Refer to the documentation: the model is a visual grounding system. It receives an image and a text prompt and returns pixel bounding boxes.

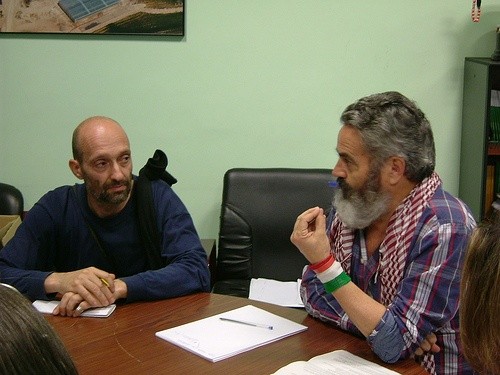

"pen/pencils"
[101,278,112,289]
[220,318,274,330]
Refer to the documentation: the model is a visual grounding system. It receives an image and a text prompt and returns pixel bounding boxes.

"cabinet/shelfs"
[460,57,500,221]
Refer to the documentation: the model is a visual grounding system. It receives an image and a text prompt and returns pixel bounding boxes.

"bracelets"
[307,253,351,294]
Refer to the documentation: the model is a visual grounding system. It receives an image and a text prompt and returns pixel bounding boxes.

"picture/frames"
[0,0,184,36]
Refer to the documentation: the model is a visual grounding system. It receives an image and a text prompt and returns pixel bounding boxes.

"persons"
[458,211,500,375]
[0,285,78,375]
[0,116,211,318]
[290,91,478,375]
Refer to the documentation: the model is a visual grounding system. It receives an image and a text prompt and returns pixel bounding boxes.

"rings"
[76,306,84,313]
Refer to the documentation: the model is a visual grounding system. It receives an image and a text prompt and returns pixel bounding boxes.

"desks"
[42,292,433,375]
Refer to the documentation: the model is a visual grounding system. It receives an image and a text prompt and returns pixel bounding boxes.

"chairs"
[199,167,338,298]
